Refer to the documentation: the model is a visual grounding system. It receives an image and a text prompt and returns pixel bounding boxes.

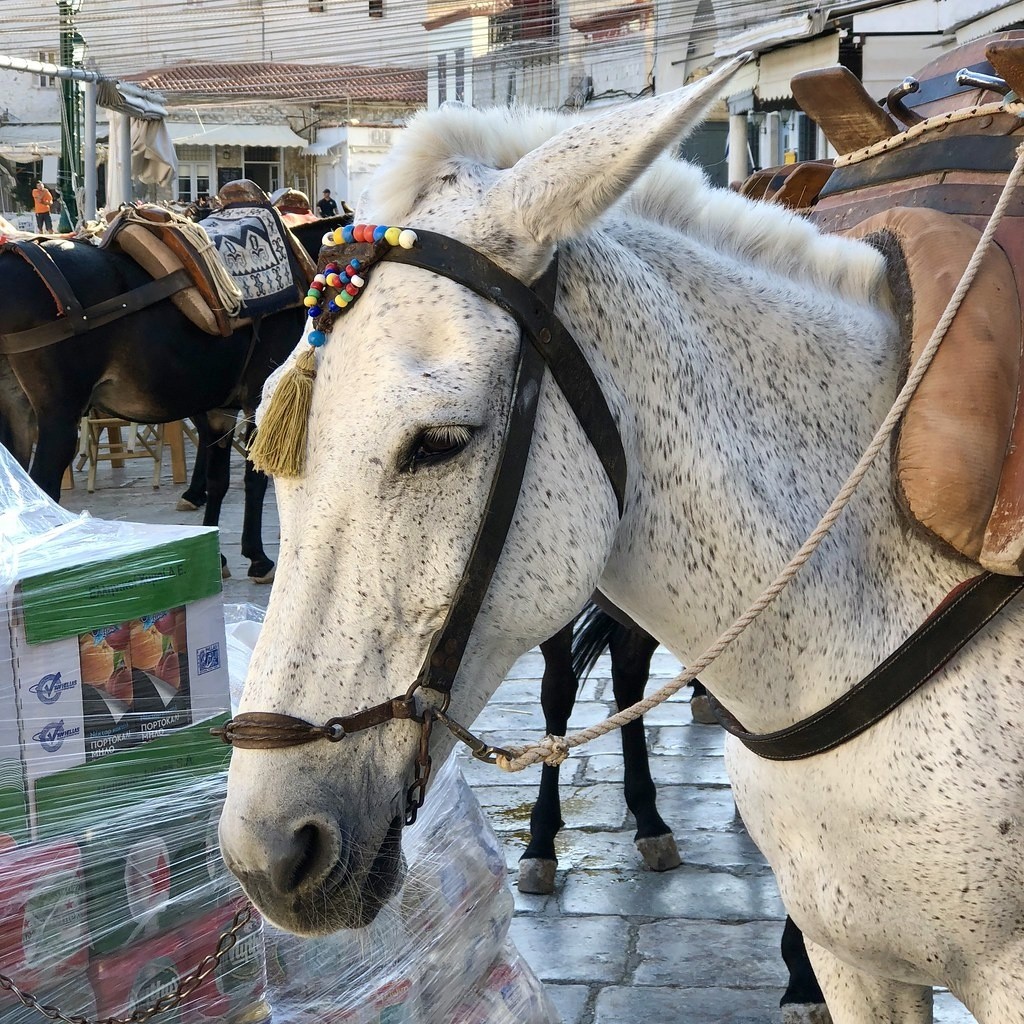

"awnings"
[300,139,346,158]
[164,122,308,150]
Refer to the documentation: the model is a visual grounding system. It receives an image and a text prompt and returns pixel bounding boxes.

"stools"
[78,405,168,493]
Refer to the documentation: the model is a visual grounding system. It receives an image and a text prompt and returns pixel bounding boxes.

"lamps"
[223,145,232,160]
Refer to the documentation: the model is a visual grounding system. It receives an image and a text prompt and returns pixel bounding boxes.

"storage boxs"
[0,521,271,1024]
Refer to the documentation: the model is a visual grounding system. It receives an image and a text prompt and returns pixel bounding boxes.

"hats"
[323,189,330,193]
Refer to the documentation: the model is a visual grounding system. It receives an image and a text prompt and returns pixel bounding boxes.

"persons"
[32,180,53,235]
[317,189,339,219]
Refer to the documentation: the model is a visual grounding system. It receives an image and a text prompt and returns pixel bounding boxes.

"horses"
[215,50,1024,1024]
[1,201,834,1023]
